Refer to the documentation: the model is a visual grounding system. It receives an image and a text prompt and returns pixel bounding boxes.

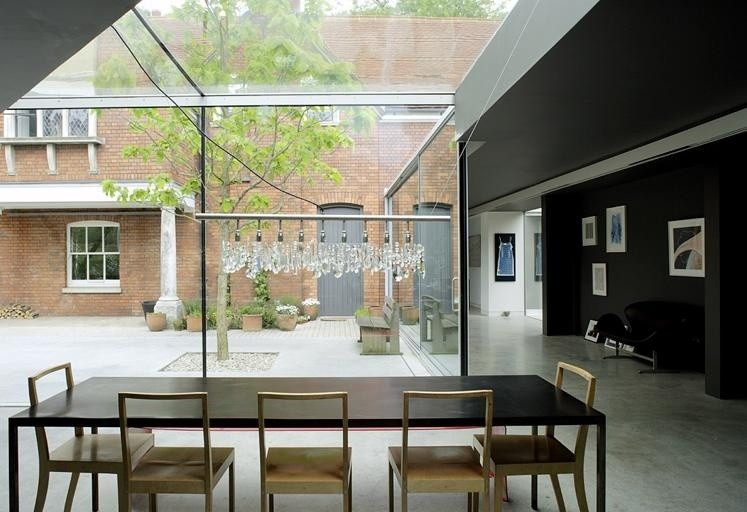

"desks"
[8,375,606,512]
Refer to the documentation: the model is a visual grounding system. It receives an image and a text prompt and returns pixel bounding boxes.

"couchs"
[597,300,705,374]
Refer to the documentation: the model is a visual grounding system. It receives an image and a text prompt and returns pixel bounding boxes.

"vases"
[402,305,418,325]
[276,314,298,331]
[146,312,166,331]
[142,301,157,321]
[303,305,320,320]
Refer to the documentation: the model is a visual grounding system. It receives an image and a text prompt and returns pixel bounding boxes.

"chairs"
[28,362,154,512]
[257,391,352,512]
[388,390,494,512]
[473,362,596,512]
[118,391,235,512]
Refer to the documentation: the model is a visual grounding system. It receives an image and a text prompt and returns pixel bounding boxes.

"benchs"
[420,295,458,354]
[357,296,403,355]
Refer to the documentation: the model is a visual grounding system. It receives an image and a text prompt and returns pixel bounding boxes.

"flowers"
[276,304,300,315]
[301,298,320,306]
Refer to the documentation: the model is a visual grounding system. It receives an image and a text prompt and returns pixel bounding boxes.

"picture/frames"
[584,320,635,353]
[468,234,481,267]
[495,233,516,281]
[581,205,628,296]
[534,232,543,281]
[668,218,705,278]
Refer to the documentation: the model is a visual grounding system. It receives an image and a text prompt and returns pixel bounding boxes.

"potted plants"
[172,300,273,332]
